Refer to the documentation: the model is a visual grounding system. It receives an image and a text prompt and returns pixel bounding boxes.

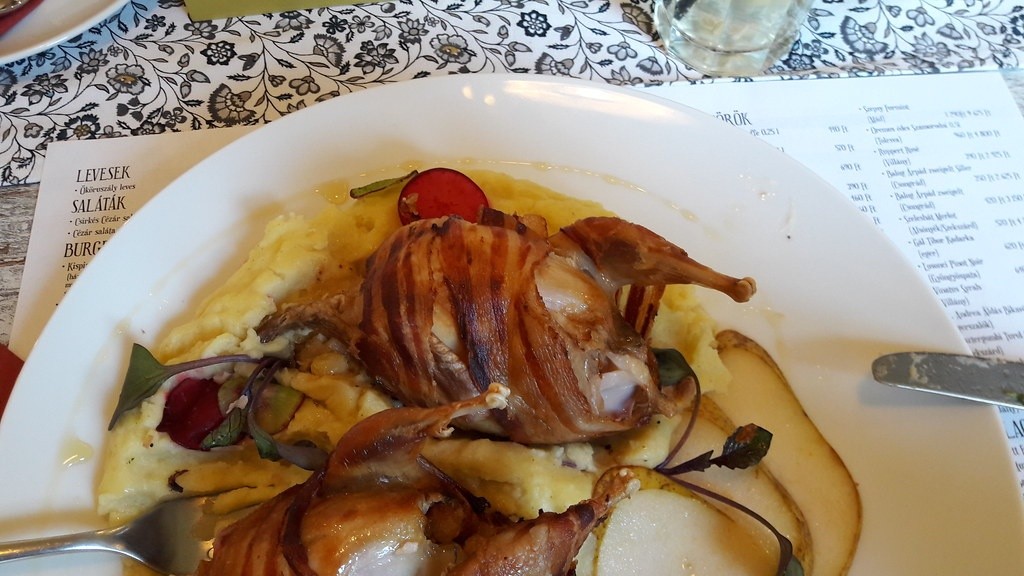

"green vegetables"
[649,345,805,576]
[105,345,294,461]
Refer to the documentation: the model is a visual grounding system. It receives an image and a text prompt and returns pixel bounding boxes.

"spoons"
[0,495,234,572]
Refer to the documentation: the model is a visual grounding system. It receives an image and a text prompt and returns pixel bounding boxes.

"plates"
[0,73,1024,576]
[0,0,131,69]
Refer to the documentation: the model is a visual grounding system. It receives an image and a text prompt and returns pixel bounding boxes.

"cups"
[650,0,813,77]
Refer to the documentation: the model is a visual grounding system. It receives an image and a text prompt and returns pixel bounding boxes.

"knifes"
[869,349,1024,413]
